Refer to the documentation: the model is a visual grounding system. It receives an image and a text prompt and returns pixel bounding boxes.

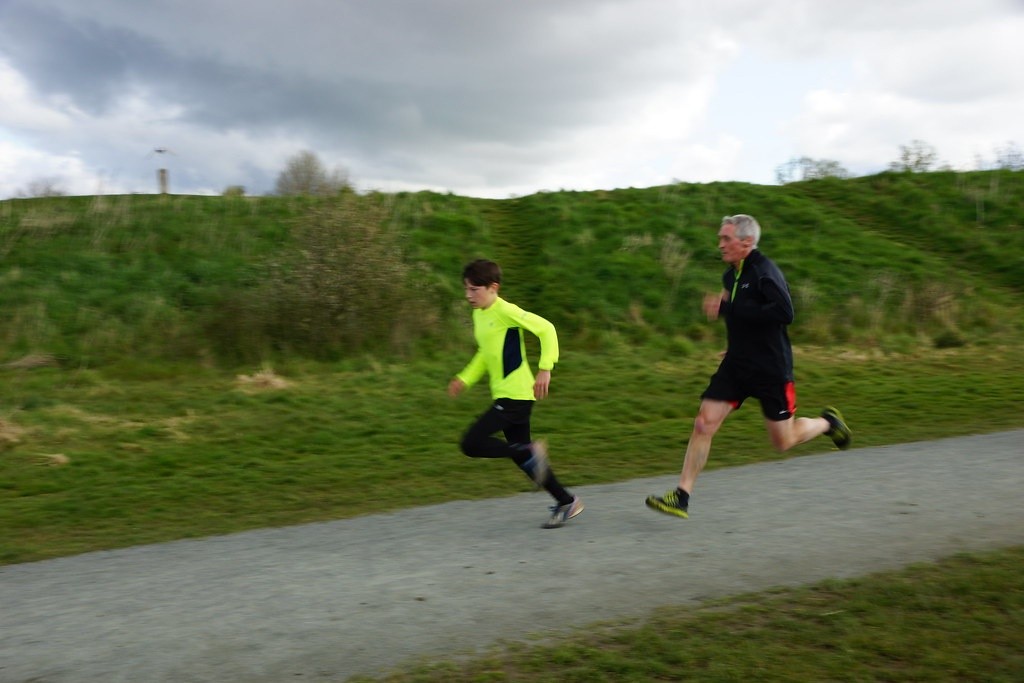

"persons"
[645,214,851,519]
[446,259,583,529]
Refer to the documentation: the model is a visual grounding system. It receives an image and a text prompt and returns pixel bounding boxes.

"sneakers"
[821,405,851,451]
[646,489,690,519]
[517,439,546,484]
[542,494,584,526]
